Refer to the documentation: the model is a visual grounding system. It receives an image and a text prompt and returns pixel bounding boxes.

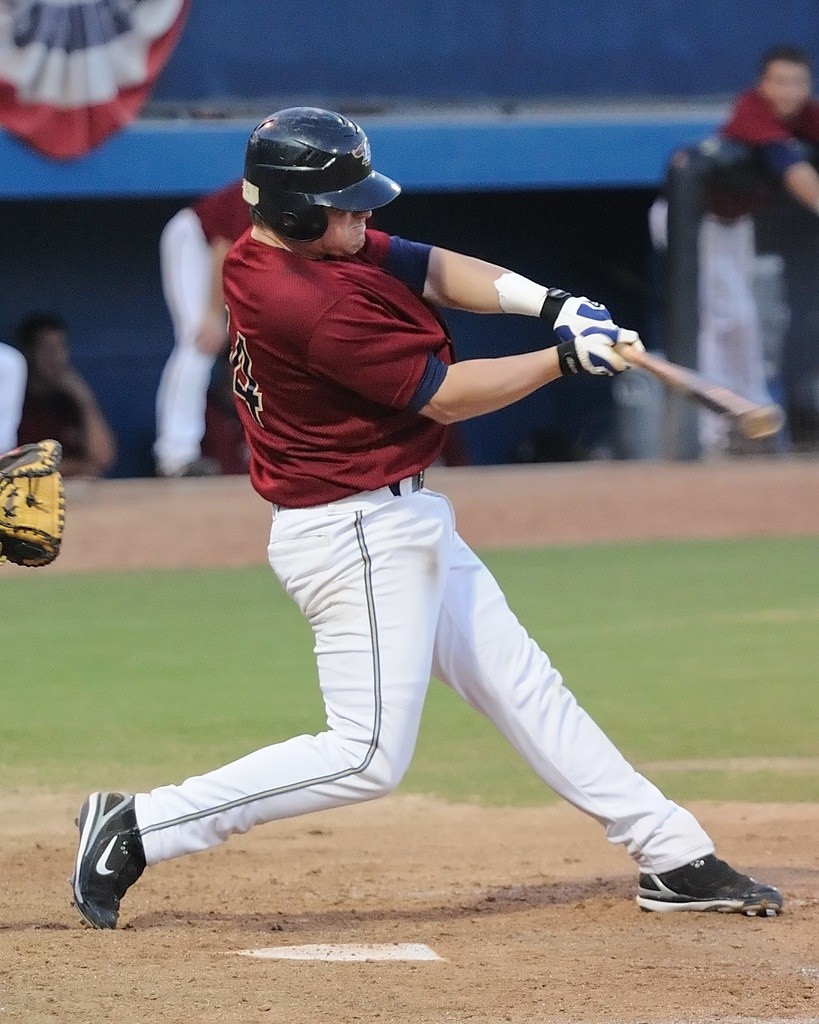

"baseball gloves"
[1,437,70,568]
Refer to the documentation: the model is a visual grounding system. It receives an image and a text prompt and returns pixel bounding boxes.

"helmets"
[241,106,403,242]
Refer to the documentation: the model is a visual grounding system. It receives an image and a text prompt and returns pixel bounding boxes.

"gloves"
[539,287,620,342]
[556,324,647,380]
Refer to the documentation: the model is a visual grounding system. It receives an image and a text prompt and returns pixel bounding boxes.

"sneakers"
[67,790,146,929]
[635,853,786,917]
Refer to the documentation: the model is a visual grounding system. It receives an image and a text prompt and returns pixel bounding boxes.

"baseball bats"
[613,337,786,440]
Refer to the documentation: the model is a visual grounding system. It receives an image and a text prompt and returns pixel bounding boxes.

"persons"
[12,322,118,488]
[72,105,786,944]
[709,48,819,298]
